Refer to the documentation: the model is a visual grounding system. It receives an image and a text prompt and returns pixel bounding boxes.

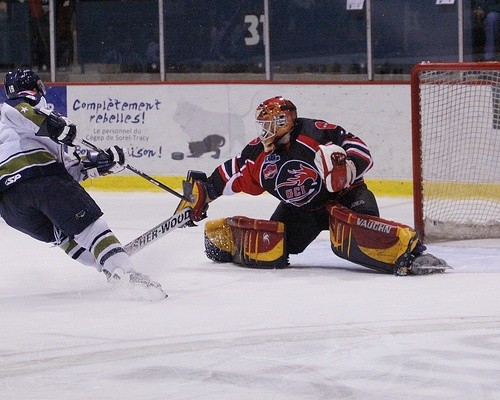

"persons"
[173,94,454,276]
[0,68,170,302]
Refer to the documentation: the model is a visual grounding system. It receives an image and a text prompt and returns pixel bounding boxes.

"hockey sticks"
[31,106,194,204]
[123,207,192,257]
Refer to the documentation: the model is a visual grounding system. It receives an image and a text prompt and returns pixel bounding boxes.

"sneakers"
[102,269,168,301]
[409,253,453,275]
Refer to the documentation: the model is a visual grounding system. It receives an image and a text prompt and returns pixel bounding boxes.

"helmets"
[4,67,47,101]
[255,96,297,139]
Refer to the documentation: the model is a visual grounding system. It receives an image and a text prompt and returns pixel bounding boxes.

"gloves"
[35,109,77,145]
[80,145,128,181]
